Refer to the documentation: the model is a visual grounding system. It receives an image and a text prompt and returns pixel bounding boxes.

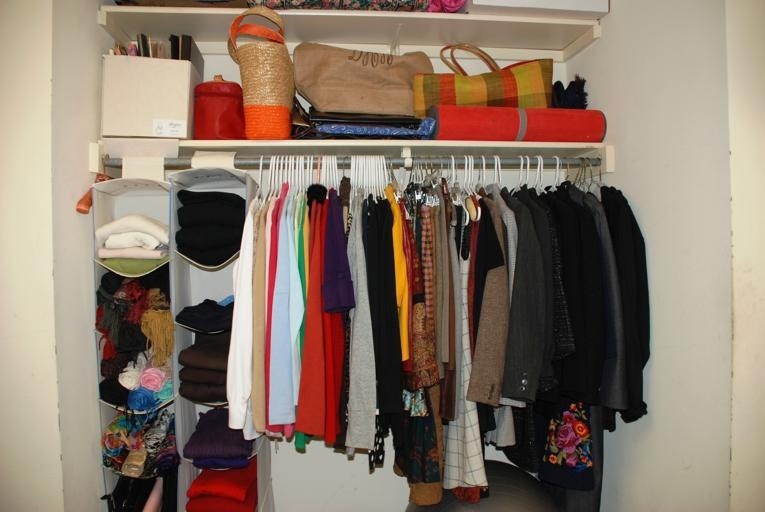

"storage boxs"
[102,56,203,138]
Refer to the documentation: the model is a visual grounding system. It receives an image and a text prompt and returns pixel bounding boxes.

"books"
[108,28,204,80]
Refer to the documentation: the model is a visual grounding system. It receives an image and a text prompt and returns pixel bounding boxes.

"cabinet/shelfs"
[88,1,615,195]
[90,167,275,511]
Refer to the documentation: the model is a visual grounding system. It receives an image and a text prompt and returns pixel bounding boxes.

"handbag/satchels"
[234,43,295,139]
[414,43,553,119]
[192,74,243,140]
